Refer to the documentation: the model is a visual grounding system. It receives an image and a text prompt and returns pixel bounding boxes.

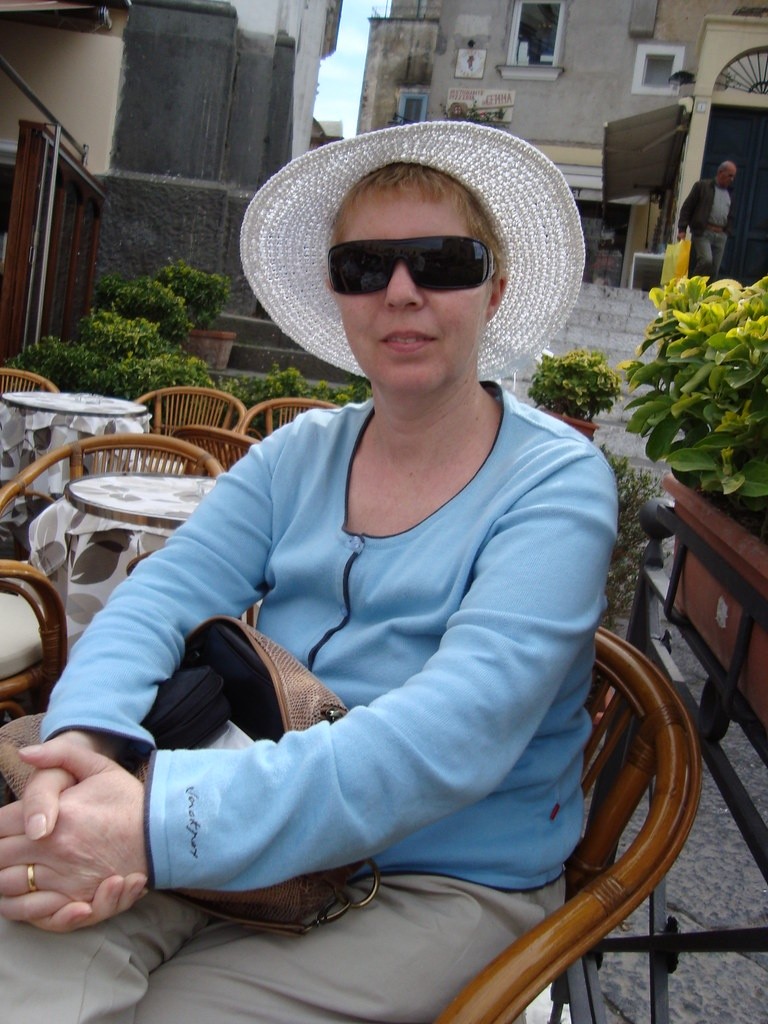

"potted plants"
[527,347,623,441]
[154,256,237,372]
[620,226,768,728]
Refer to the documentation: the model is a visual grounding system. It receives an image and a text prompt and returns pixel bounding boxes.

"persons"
[674,161,736,284]
[0,121,619,1023]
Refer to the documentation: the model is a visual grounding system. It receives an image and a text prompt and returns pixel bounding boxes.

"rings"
[27,864,37,891]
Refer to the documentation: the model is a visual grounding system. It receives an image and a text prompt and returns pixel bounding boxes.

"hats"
[238,121,585,385]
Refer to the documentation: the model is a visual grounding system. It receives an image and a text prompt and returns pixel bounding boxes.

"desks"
[29,471,264,658]
[0,392,153,561]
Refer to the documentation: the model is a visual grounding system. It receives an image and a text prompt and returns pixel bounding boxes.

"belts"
[708,225,724,231]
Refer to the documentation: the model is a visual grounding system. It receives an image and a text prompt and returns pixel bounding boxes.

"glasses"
[324,235,494,296]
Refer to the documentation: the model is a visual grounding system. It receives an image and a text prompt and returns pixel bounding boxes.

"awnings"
[602,94,694,204]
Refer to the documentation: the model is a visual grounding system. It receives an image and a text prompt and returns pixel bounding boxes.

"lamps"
[668,71,696,86]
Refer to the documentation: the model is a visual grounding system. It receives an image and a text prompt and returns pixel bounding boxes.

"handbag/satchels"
[0,616,378,937]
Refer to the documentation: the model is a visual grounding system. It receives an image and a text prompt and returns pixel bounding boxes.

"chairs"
[0,367,61,400]
[432,628,702,1024]
[171,425,261,478]
[0,558,70,729]
[235,398,341,463]
[0,432,225,565]
[131,386,248,473]
